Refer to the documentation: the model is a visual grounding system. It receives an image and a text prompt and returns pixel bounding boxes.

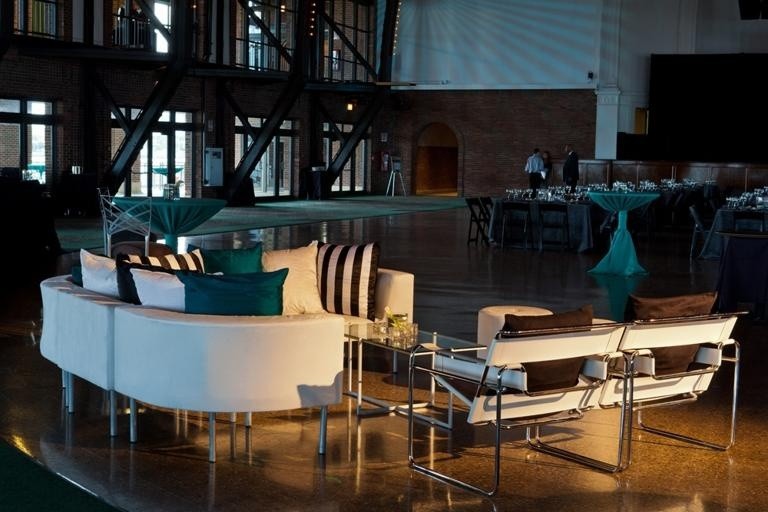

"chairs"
[536,310,751,472]
[409,310,630,497]
[464,196,492,244]
[478,193,495,238]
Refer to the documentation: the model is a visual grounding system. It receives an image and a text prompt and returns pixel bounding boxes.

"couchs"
[40,267,414,464]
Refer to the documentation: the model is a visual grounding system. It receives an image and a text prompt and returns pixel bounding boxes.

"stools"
[477,304,554,362]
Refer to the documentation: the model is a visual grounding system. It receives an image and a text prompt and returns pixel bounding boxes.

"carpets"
[1,429,130,512]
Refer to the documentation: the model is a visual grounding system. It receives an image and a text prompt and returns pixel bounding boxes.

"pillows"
[499,304,594,394]
[624,290,718,377]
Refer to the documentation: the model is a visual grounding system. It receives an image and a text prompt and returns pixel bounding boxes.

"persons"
[524,148,545,197]
[562,143,580,193]
[542,150,552,180]
